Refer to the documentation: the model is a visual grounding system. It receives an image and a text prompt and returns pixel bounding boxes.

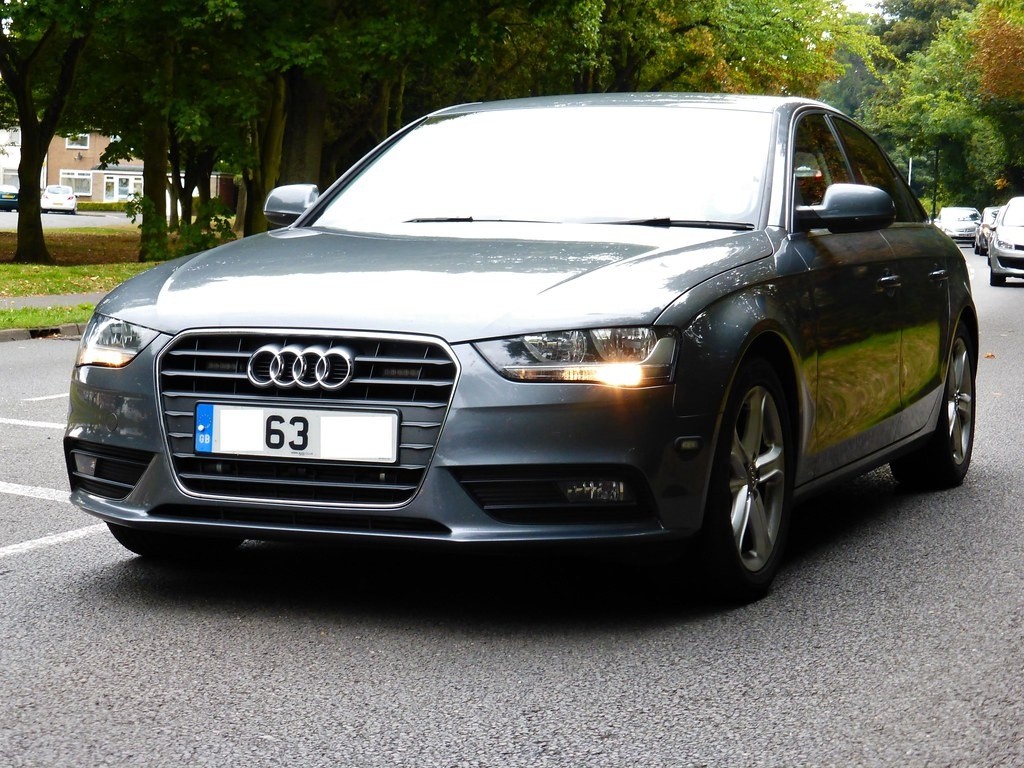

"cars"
[0,185,20,212]
[40,185,79,216]
[983,196,1024,287]
[974,205,1004,257]
[928,206,982,248]
[63,92,977,616]
[794,166,821,179]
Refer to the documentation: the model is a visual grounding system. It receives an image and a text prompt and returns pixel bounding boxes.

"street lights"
[907,136,918,188]
